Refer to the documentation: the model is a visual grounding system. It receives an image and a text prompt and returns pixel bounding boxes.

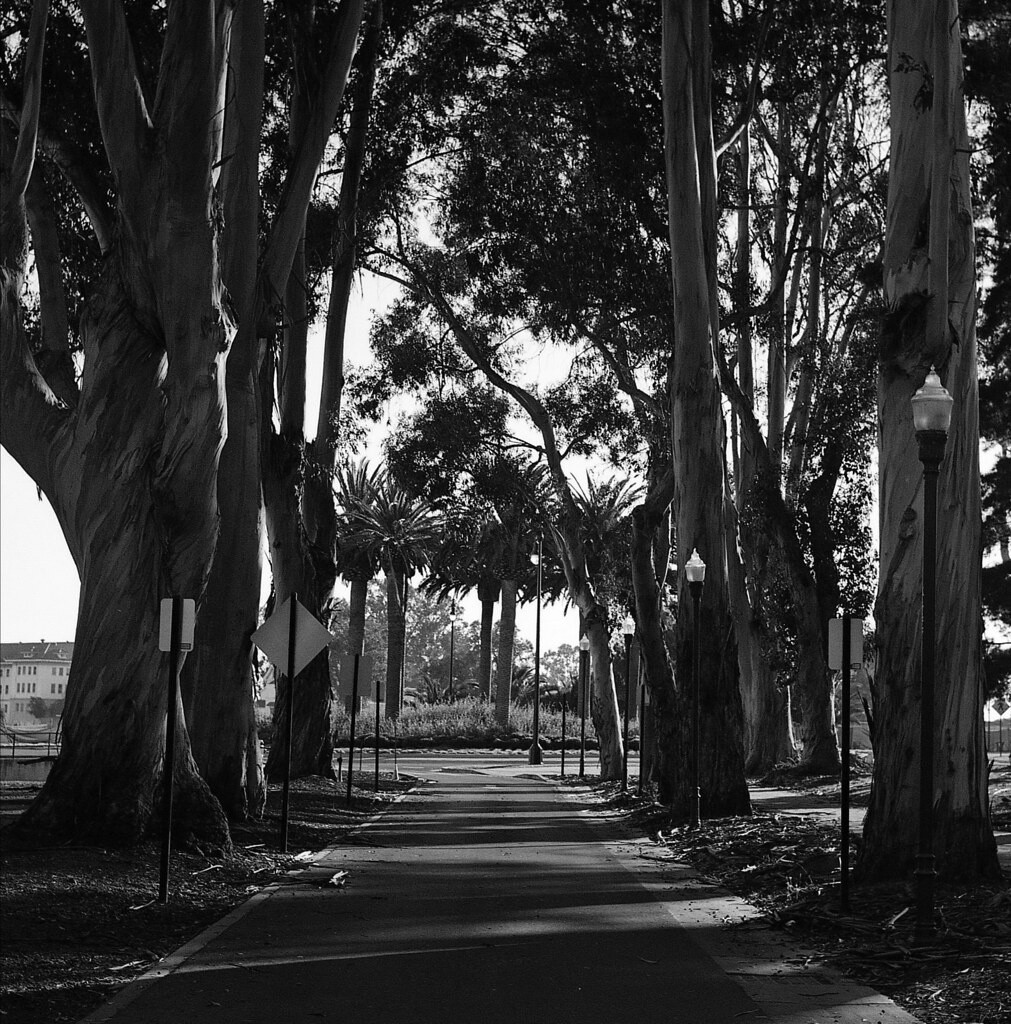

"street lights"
[578,632,591,777]
[450,597,457,693]
[684,547,708,830]
[906,364,956,966]
[529,531,543,765]
